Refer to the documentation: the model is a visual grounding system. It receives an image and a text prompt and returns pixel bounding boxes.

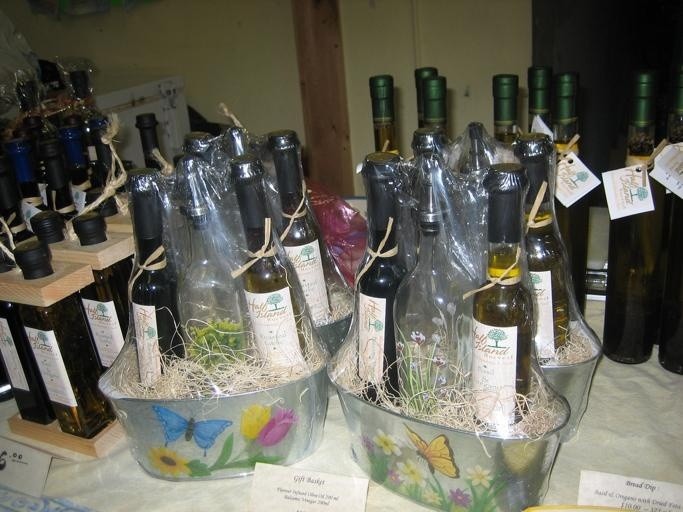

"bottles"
[1,68,683,438]
